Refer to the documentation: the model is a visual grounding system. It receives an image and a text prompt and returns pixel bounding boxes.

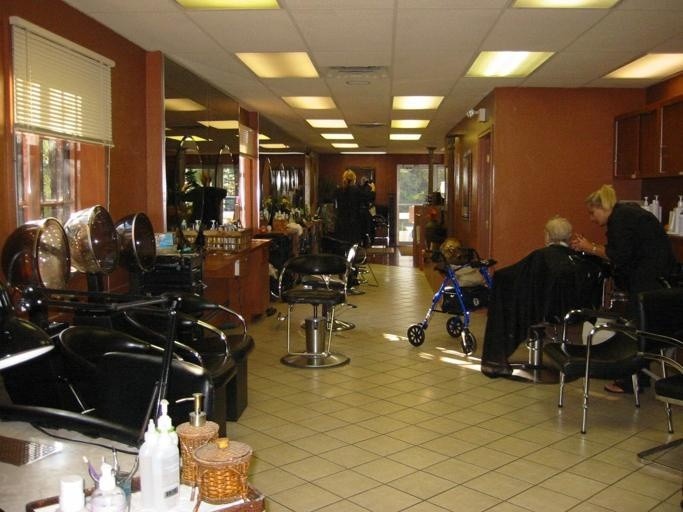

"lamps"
[466,108,486,122]
[0,282,56,370]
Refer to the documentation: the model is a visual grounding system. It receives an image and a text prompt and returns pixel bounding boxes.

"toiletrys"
[153,422,179,509]
[649,194,662,225]
[641,196,650,213]
[176,393,219,485]
[139,420,160,509]
[674,194,683,234]
[91,463,126,512]
[157,398,179,446]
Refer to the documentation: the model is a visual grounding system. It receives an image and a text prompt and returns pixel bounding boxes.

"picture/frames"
[461,152,472,224]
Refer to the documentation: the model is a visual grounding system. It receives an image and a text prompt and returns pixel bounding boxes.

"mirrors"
[164,55,252,235]
[259,113,306,226]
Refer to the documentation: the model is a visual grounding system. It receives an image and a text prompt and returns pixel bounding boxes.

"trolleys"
[406,243,498,355]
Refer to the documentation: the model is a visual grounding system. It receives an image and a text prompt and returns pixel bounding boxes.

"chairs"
[373,207,390,247]
[60,323,183,394]
[634,286,683,434]
[542,272,672,434]
[356,233,380,288]
[120,307,240,437]
[160,289,255,421]
[80,351,215,448]
[278,252,351,369]
[506,262,610,384]
[299,236,360,332]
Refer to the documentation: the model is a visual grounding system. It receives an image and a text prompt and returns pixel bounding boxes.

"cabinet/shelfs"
[202,237,274,331]
[612,94,683,180]
[258,218,324,268]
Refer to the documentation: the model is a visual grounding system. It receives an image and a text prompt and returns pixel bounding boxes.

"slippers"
[604,382,643,394]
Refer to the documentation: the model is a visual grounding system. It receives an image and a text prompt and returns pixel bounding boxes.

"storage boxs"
[441,286,485,313]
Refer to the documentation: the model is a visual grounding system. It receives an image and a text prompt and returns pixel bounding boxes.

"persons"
[491,216,591,293]
[334,168,376,246]
[571,184,673,394]
